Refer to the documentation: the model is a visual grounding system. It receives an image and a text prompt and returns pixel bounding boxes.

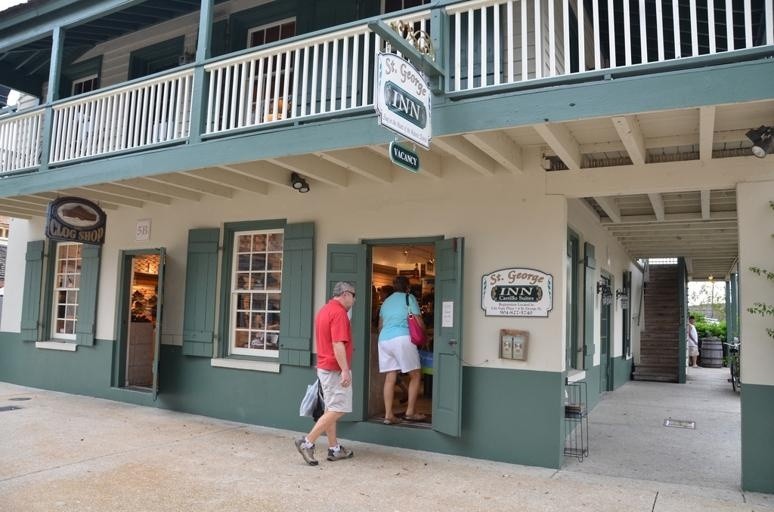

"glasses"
[345,290,355,298]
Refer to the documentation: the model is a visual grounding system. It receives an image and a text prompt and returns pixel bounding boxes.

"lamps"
[595,280,613,306]
[290,170,309,194]
[615,287,629,310]
[744,123,773,159]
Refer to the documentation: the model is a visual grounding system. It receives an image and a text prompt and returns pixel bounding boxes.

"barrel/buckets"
[700,337,724,368]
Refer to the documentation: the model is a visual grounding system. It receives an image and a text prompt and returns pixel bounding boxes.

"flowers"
[130,288,156,316]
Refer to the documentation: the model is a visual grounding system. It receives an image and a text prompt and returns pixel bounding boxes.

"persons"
[292,280,356,466]
[373,285,412,403]
[149,285,159,371]
[688,315,700,369]
[375,272,428,425]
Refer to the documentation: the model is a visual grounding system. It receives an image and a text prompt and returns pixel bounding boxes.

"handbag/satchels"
[312,393,325,422]
[408,312,427,346]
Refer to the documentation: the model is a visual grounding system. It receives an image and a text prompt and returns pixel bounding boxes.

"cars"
[704,318,720,326]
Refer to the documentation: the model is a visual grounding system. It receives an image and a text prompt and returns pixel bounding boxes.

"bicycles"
[723,337,740,392]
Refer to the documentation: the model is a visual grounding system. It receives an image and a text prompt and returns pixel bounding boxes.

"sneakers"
[295,436,319,466]
[327,444,353,461]
